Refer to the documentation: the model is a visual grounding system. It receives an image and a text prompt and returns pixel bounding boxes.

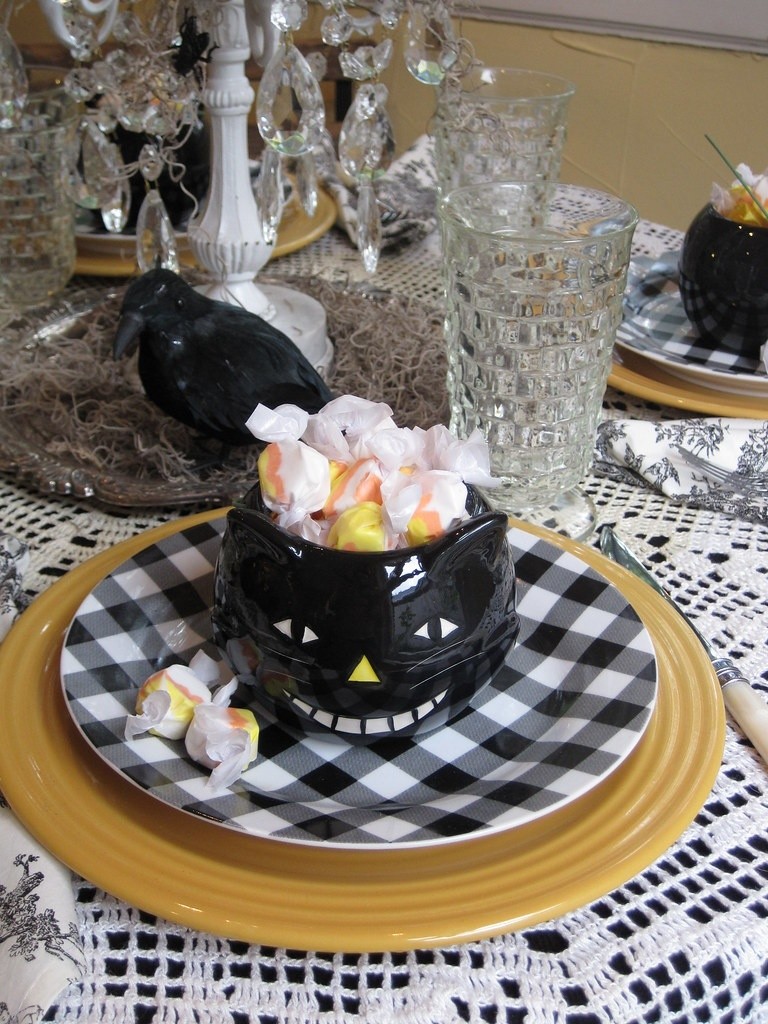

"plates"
[59,515,660,851]
[614,249,768,398]
[71,159,293,253]
[71,171,339,276]
[0,504,727,953]
[606,339,768,419]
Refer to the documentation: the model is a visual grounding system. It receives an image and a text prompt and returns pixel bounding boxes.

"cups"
[433,66,576,196]
[439,180,638,547]
[0,101,82,303]
[0,63,77,101]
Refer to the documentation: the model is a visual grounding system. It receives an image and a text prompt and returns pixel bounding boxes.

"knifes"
[599,525,767,765]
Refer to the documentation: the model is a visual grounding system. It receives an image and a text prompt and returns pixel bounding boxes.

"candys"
[710,162,768,229]
[244,393,501,552]
[121,649,259,790]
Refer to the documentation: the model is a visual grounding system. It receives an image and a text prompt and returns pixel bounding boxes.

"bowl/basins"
[211,478,520,742]
[677,203,768,359]
[84,128,212,234]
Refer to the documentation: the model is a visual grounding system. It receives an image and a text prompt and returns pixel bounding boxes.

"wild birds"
[110,267,336,480]
